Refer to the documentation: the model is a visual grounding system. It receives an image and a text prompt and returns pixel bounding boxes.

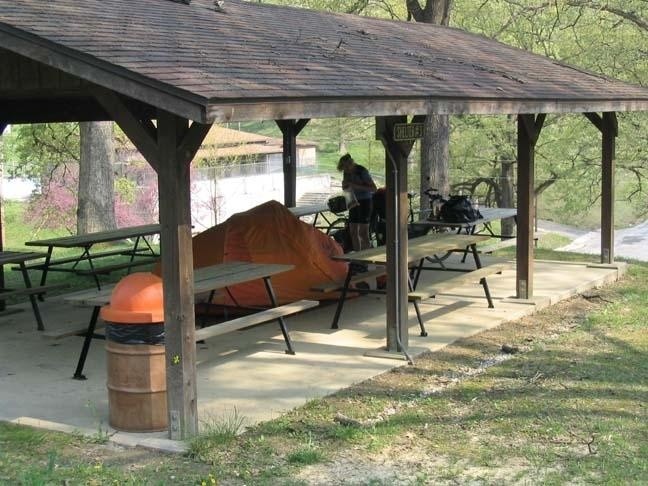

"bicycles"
[323,186,458,266]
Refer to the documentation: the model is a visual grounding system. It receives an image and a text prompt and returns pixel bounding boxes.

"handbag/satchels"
[441,195,483,223]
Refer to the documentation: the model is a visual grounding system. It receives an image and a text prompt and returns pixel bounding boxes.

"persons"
[337,153,378,274]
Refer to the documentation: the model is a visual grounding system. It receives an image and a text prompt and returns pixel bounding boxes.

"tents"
[152,199,359,310]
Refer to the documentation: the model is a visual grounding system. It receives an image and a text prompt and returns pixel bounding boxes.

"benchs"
[310,233,513,337]
[47,260,320,380]
[2,248,70,331]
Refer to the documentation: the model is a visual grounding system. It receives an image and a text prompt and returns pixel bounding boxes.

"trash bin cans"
[98,271,169,433]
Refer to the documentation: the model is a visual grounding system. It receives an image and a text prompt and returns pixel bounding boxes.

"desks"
[411,207,517,228]
[26,224,194,301]
[288,204,334,226]
